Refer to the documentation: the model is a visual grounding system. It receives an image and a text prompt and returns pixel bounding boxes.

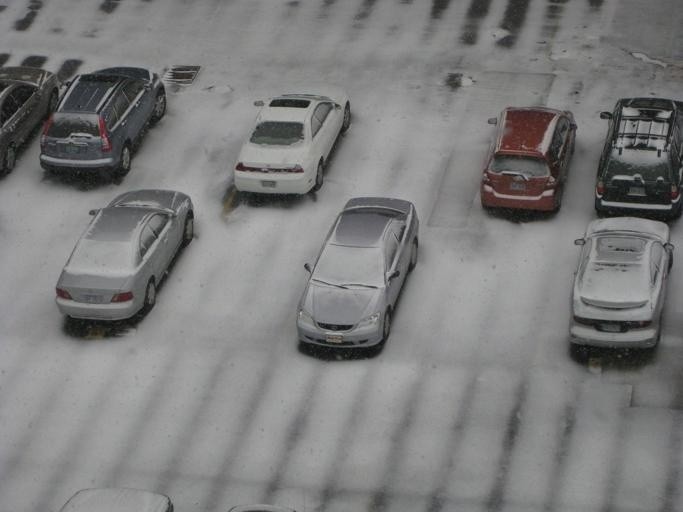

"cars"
[55,190,194,321]
[570,98,683,349]
[0,67,166,176]
[59,488,173,511]
[480,107,577,211]
[234,84,350,194]
[296,197,419,348]
